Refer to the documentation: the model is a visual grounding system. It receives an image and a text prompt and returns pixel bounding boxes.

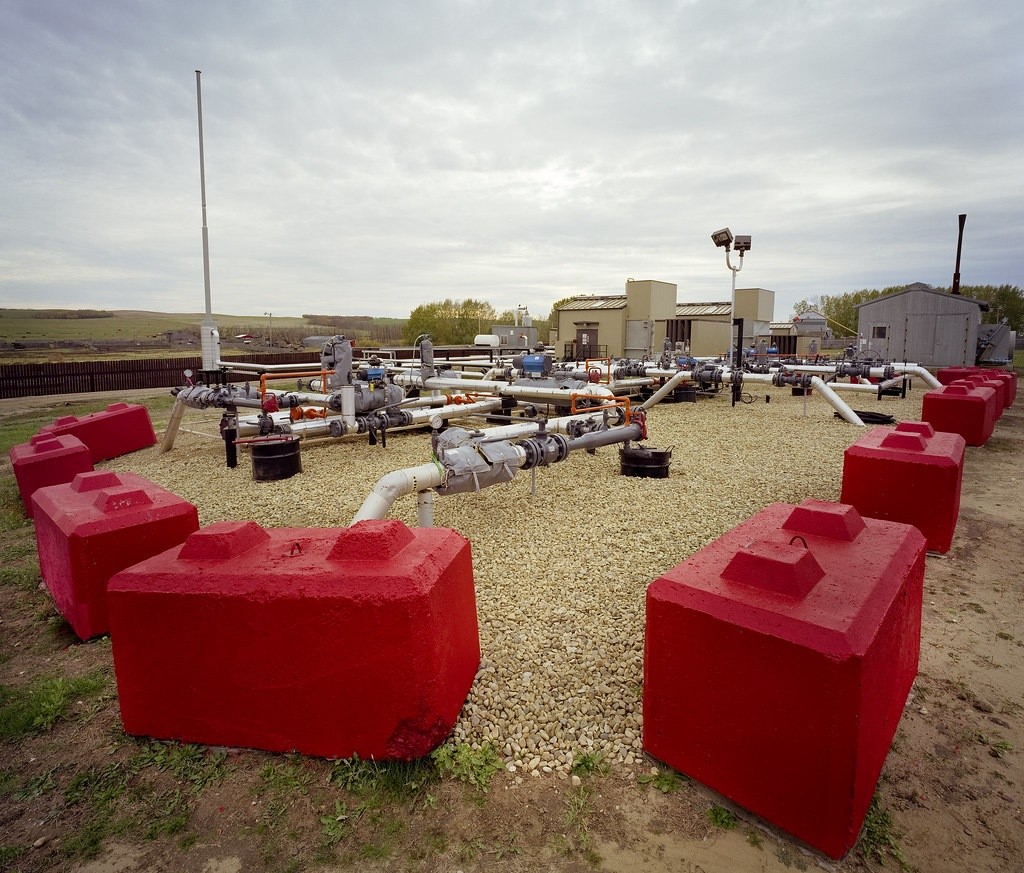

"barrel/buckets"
[474,334,499,347]
[248,433,303,480]
[792,387,813,396]
[619,443,676,478]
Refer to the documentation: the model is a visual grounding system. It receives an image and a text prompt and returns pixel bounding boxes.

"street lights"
[710,226,752,404]
